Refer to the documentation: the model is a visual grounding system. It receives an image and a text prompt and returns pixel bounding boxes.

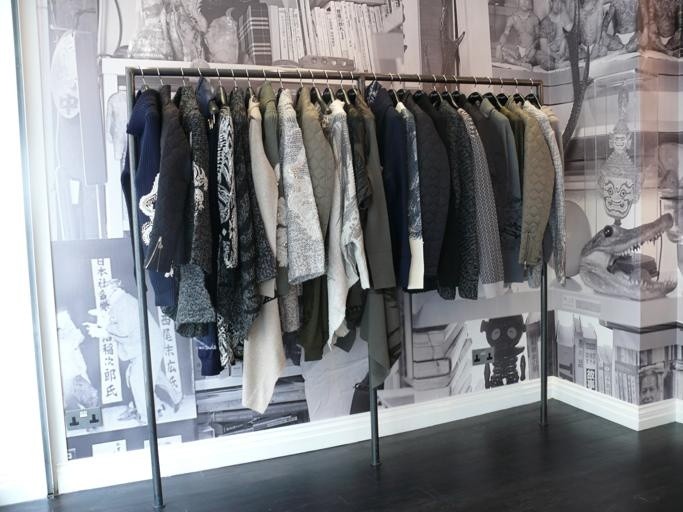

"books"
[267,0,406,75]
[375,324,474,408]
[193,364,309,441]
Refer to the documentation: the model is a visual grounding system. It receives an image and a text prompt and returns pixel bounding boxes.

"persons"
[56,306,98,413]
[494,0,683,71]
[82,278,162,423]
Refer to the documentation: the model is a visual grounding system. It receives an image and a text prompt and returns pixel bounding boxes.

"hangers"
[129,63,548,117]
